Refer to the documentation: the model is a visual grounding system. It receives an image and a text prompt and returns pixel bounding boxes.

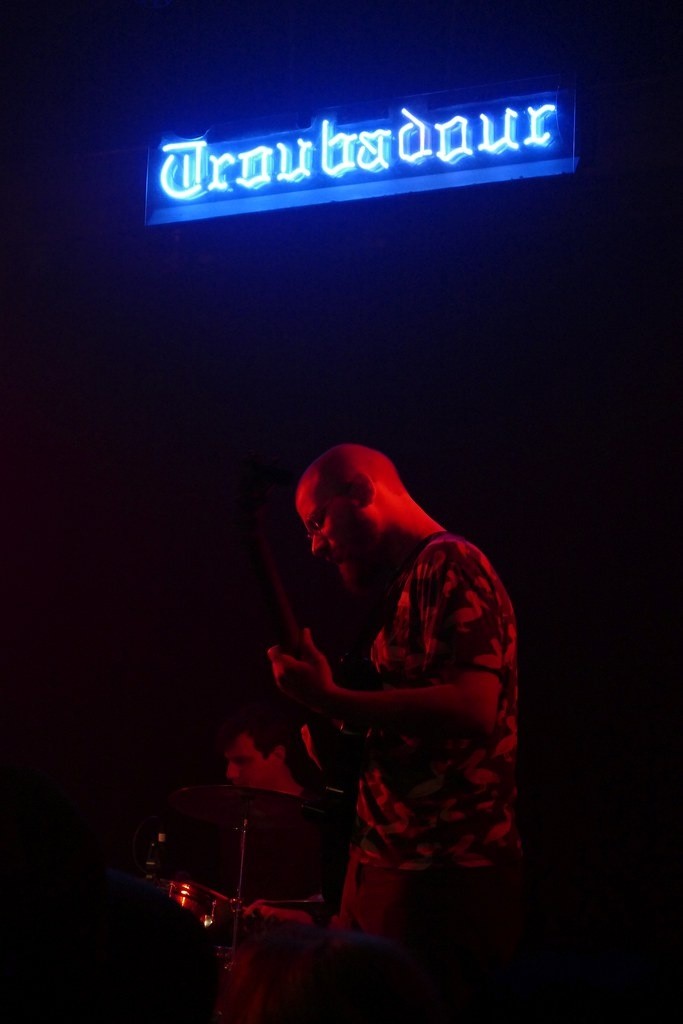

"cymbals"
[165,781,307,830]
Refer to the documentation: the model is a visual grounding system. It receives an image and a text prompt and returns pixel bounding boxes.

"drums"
[164,871,232,926]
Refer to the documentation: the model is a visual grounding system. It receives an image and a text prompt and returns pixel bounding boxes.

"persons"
[0,768,219,1024]
[217,712,321,910]
[266,442,519,936]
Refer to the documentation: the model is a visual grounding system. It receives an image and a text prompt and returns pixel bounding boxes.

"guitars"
[242,499,376,797]
